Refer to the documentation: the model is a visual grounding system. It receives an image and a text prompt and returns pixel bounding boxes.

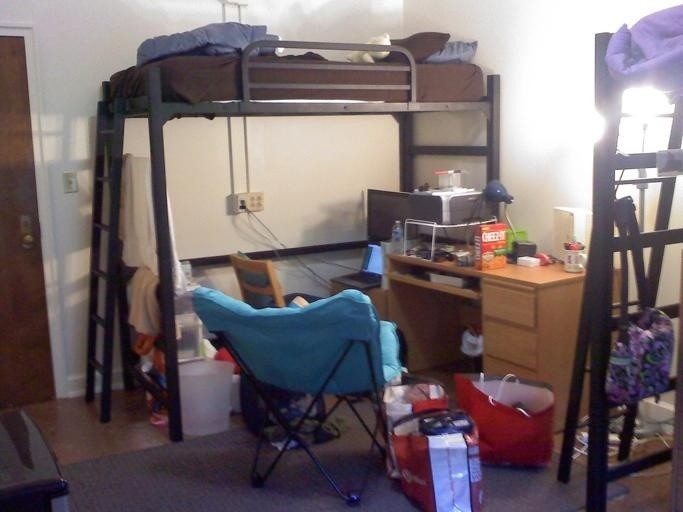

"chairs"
[227,251,321,310]
[186,286,409,507]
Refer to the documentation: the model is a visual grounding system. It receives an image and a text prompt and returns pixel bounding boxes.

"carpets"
[59,395,629,512]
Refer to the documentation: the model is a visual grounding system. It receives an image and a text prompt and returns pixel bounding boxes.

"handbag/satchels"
[604,305,675,407]
[451,371,557,469]
[389,407,489,511]
[380,376,451,482]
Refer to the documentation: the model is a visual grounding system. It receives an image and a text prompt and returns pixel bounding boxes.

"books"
[475,224,508,271]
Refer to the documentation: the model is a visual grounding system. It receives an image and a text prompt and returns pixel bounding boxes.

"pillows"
[424,40,478,64]
[382,31,450,65]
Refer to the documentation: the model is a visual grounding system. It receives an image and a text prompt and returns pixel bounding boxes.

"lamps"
[482,178,520,255]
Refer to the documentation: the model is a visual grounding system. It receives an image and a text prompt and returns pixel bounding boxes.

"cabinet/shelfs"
[125,280,206,368]
[329,268,385,323]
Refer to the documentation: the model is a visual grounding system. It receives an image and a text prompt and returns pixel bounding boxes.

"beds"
[83,55,504,442]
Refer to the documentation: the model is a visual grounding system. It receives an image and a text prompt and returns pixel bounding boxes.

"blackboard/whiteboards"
[113,114,406,266]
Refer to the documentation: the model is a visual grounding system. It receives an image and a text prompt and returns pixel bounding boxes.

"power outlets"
[231,191,250,213]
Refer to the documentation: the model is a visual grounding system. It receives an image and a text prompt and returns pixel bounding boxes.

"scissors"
[563,241,585,251]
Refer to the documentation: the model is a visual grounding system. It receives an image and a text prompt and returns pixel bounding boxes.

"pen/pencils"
[434,170,465,175]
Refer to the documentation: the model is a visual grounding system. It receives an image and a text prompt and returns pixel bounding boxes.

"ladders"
[86,97,126,423]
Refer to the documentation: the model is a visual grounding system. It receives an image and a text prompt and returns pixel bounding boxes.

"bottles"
[390,220,406,254]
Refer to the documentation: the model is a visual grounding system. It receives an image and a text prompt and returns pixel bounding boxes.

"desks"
[0,406,72,512]
[383,242,627,435]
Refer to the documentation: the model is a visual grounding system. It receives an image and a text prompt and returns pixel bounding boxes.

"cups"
[562,243,588,273]
